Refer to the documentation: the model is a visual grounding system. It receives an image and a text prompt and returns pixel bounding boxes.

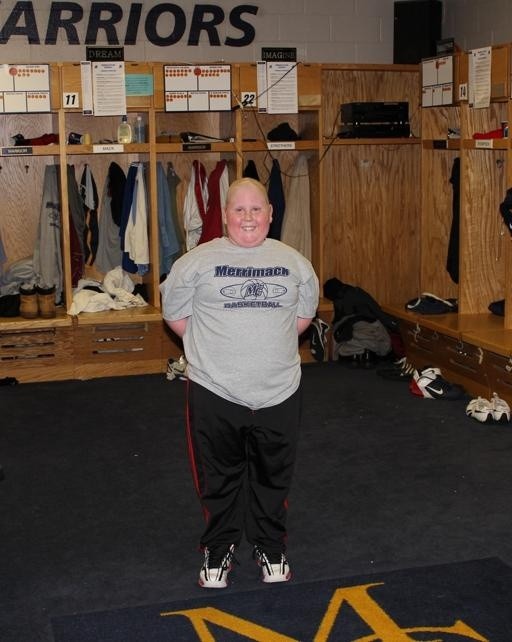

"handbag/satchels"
[404,291,458,315]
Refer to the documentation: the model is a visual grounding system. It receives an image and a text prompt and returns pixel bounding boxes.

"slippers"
[377,357,417,381]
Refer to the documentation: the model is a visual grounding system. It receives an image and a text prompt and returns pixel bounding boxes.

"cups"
[82,133,91,145]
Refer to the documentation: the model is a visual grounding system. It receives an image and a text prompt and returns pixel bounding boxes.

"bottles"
[115,113,147,143]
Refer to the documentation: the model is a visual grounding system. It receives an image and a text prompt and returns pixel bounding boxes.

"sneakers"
[339,349,374,370]
[408,367,466,400]
[197,541,235,589]
[165,354,190,382]
[466,397,494,423]
[488,392,511,423]
[252,543,293,583]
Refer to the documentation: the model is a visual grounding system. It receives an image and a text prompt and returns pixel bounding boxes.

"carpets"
[50,556,512,642]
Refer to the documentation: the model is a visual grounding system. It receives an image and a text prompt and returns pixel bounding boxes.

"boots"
[18,282,58,319]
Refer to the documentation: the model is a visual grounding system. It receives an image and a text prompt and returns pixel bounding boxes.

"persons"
[159,173,321,592]
